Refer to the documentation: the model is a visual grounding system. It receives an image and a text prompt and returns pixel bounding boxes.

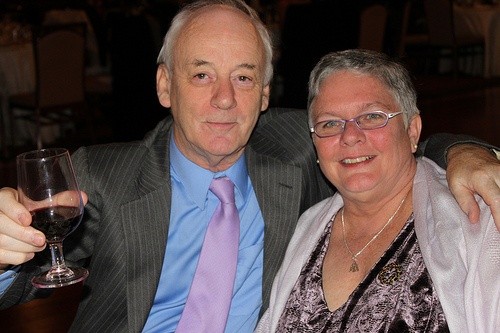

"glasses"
[310,111,402,138]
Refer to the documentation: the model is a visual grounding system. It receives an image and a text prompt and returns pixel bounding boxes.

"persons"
[254,50,500,333]
[0,0,500,333]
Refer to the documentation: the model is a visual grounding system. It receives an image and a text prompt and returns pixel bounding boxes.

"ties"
[175,177,240,333]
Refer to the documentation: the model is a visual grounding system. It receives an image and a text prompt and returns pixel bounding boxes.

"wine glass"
[16,148,90,289]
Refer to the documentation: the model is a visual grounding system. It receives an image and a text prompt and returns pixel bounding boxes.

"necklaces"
[341,196,412,274]
